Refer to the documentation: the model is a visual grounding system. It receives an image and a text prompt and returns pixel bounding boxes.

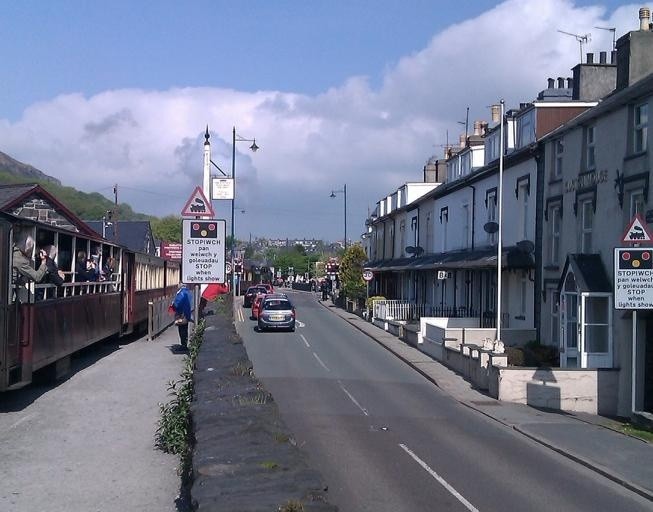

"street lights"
[229,126,258,295]
[330,182,346,309]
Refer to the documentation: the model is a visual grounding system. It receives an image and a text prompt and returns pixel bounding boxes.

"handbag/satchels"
[174,315,187,326]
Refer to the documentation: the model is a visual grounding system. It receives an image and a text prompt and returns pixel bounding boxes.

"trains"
[0,183,194,394]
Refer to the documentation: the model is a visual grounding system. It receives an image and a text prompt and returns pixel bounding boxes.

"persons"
[11,232,115,300]
[320,279,331,301]
[197,283,228,320]
[171,282,192,351]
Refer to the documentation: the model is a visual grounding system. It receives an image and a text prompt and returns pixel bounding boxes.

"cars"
[241,283,295,334]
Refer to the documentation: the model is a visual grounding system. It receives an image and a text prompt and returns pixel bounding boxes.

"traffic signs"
[362,269,373,279]
